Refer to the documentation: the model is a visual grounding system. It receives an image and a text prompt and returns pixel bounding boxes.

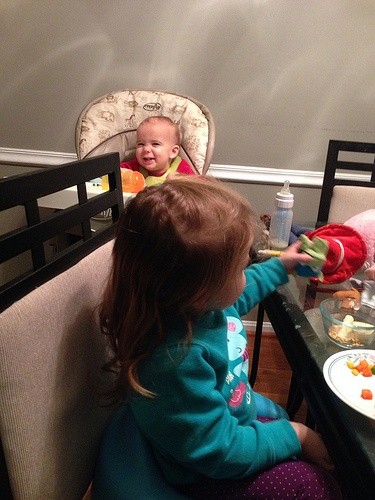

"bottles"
[268,180,294,249]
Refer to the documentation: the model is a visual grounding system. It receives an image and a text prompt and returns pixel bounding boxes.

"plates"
[323,349,375,421]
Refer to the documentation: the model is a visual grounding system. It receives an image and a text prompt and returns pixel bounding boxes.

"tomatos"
[354,360,372,376]
[362,389,372,399]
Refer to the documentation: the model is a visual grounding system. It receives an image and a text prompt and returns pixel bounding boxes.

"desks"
[248,233,375,499]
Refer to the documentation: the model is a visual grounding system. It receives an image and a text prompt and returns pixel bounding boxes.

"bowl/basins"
[320,297,375,350]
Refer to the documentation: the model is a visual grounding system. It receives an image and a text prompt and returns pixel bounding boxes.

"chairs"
[285,137,375,421]
[0,151,125,499]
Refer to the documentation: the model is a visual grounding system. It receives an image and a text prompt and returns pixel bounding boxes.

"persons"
[295,206,375,281]
[118,116,194,192]
[92,180,333,500]
